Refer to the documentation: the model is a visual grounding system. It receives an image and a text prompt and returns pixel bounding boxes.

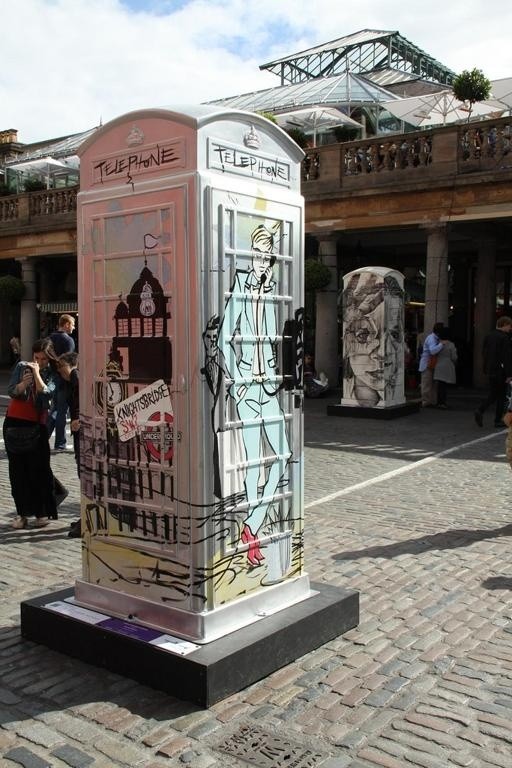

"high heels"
[241,526,265,571]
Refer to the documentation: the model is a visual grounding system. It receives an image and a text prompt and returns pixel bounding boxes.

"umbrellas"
[269,106,366,138]
[378,83,503,127]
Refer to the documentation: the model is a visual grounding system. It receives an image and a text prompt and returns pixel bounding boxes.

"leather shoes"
[55,443,64,450]
[494,421,504,426]
[474,409,483,427]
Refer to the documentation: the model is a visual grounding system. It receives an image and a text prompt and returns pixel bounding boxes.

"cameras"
[24,365,31,374]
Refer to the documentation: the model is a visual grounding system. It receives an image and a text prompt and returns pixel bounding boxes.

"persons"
[473,315,511,428]
[414,324,428,389]
[2,340,69,528]
[432,326,459,405]
[340,273,405,406]
[417,321,448,406]
[215,221,297,569]
[303,351,316,395]
[58,351,82,539]
[48,314,76,450]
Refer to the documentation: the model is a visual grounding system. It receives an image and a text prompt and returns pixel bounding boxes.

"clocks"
[100,375,124,412]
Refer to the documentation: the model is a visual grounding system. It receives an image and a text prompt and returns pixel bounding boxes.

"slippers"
[12,516,26,529]
[34,516,48,527]
